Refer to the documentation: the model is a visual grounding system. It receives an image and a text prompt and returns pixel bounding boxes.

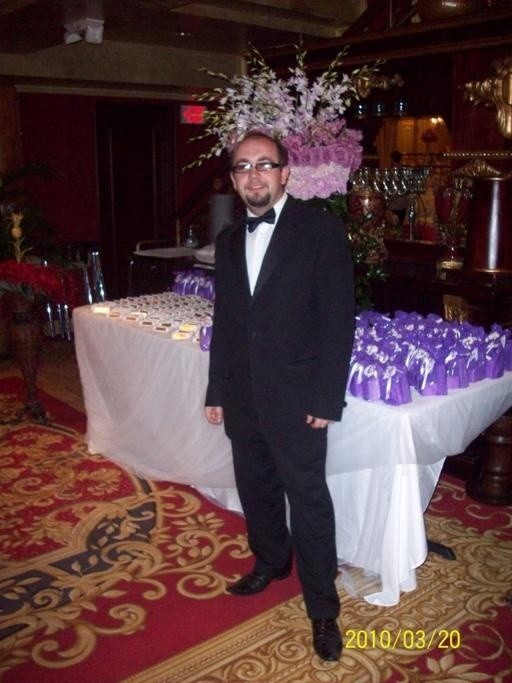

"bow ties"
[247,208,275,233]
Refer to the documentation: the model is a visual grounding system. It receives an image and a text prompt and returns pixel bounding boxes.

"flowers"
[0,206,77,314]
[174,31,406,221]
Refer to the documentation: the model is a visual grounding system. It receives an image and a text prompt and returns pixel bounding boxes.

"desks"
[40,257,92,317]
[70,292,512,609]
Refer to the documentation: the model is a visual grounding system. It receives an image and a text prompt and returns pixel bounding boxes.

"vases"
[436,172,474,270]
[345,178,390,279]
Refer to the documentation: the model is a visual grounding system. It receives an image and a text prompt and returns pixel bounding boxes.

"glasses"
[230,162,281,173]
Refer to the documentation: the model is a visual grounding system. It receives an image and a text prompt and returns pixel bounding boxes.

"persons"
[203,132,357,659]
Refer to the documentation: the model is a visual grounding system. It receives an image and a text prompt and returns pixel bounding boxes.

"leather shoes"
[227,566,290,595]
[312,618,342,661]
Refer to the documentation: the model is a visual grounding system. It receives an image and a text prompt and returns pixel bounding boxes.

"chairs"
[66,242,107,304]
